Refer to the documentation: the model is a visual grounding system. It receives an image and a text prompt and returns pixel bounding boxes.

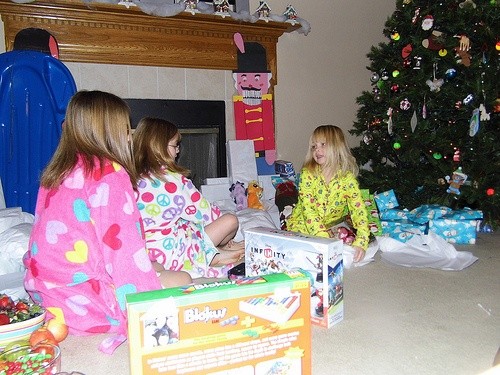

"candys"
[0,352,58,375]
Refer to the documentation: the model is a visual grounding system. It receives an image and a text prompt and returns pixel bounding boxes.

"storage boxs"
[126,140,484,375]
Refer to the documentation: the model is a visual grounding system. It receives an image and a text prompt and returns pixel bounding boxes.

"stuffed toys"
[229,181,248,212]
[245,180,264,209]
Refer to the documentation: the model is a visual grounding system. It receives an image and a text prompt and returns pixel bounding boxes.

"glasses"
[167,144,179,153]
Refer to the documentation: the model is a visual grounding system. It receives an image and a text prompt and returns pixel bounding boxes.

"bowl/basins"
[0,306,47,351]
[0,344,61,375]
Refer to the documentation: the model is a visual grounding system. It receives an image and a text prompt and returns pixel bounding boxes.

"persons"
[22,91,192,354]
[284,125,370,263]
[131,117,245,279]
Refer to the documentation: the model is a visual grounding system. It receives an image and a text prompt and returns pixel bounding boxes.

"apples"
[0,319,68,362]
[0,297,29,324]
